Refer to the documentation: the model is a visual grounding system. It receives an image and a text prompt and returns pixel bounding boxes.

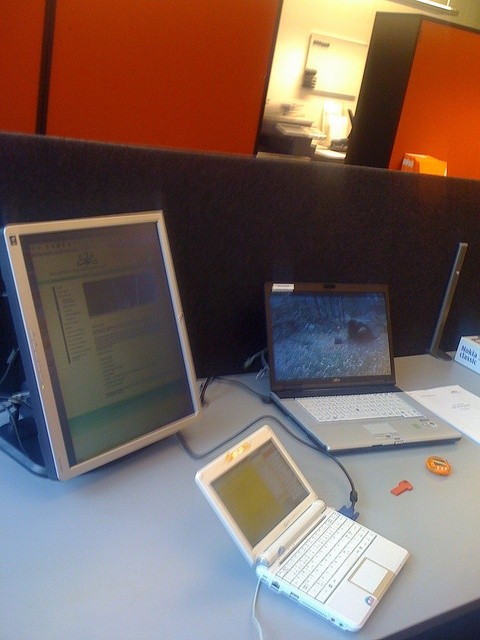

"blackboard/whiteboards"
[302,31,370,99]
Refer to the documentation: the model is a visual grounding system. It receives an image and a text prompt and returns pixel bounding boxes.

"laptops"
[194,426,411,632]
[262,281,462,453]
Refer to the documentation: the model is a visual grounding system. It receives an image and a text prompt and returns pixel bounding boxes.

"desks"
[0,348,479,640]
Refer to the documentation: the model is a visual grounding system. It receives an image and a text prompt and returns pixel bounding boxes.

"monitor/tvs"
[0,210,204,481]
